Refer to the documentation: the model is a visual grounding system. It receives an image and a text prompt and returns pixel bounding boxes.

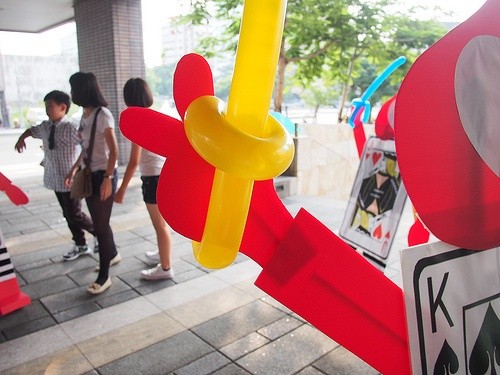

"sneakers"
[93,237,99,253]
[63,245,90,261]
[145,250,160,262]
[141,263,174,279]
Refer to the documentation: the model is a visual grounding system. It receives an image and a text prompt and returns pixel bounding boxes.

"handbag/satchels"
[70,167,92,200]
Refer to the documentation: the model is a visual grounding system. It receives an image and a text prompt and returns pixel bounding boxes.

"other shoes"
[95,252,120,270]
[86,276,112,294]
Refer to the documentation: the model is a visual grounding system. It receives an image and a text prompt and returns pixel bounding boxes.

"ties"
[48,126,56,150]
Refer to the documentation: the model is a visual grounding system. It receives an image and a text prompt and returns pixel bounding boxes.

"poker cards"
[397,240,500,375]
[336,135,409,272]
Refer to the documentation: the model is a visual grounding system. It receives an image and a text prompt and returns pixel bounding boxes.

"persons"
[64,71,120,294]
[114,78,173,278]
[15,90,99,261]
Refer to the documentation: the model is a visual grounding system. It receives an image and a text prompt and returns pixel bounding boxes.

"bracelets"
[104,175,114,179]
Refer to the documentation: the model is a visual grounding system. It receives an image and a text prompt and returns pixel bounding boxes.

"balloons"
[348,56,407,129]
[185,0,295,269]
